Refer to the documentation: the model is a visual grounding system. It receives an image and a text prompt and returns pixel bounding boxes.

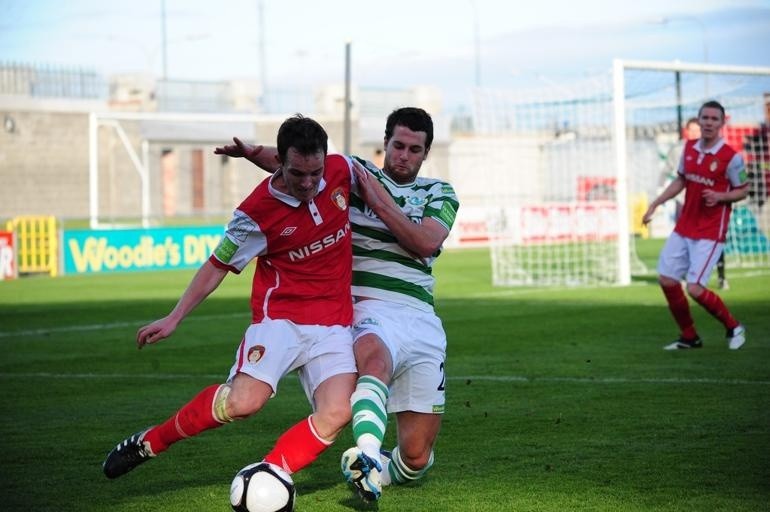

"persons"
[656,118,729,290]
[213,107,459,501]
[643,101,751,350]
[103,113,358,481]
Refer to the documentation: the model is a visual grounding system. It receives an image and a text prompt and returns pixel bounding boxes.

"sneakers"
[726,325,747,350]
[663,336,703,350]
[102,424,160,480]
[340,446,393,503]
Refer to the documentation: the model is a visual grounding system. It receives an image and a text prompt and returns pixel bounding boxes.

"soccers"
[228,461,297,512]
